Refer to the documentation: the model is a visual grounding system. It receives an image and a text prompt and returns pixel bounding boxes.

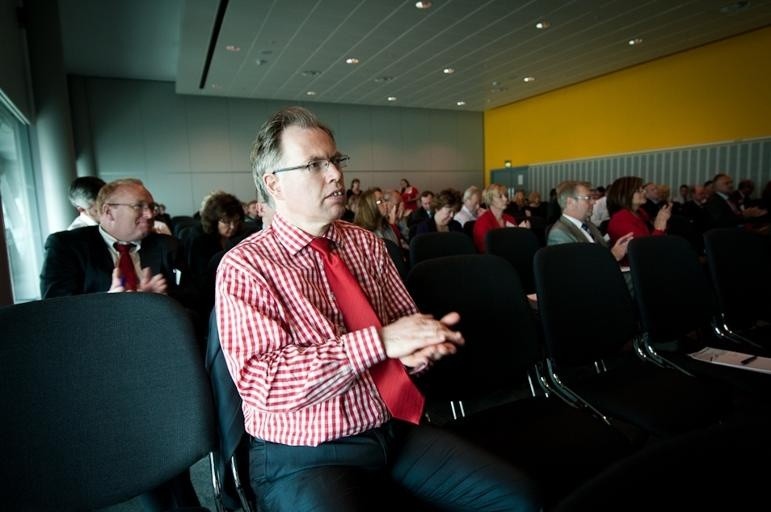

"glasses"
[271,153,351,178]
[572,195,594,200]
[107,202,161,212]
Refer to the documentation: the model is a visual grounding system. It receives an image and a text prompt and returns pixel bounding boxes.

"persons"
[40,174,766,294]
[211,104,538,512]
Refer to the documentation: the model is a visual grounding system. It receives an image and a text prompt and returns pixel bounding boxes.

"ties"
[306,237,426,426]
[114,242,141,291]
[582,223,600,244]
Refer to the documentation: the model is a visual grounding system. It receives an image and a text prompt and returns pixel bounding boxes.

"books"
[687,346,771,375]
